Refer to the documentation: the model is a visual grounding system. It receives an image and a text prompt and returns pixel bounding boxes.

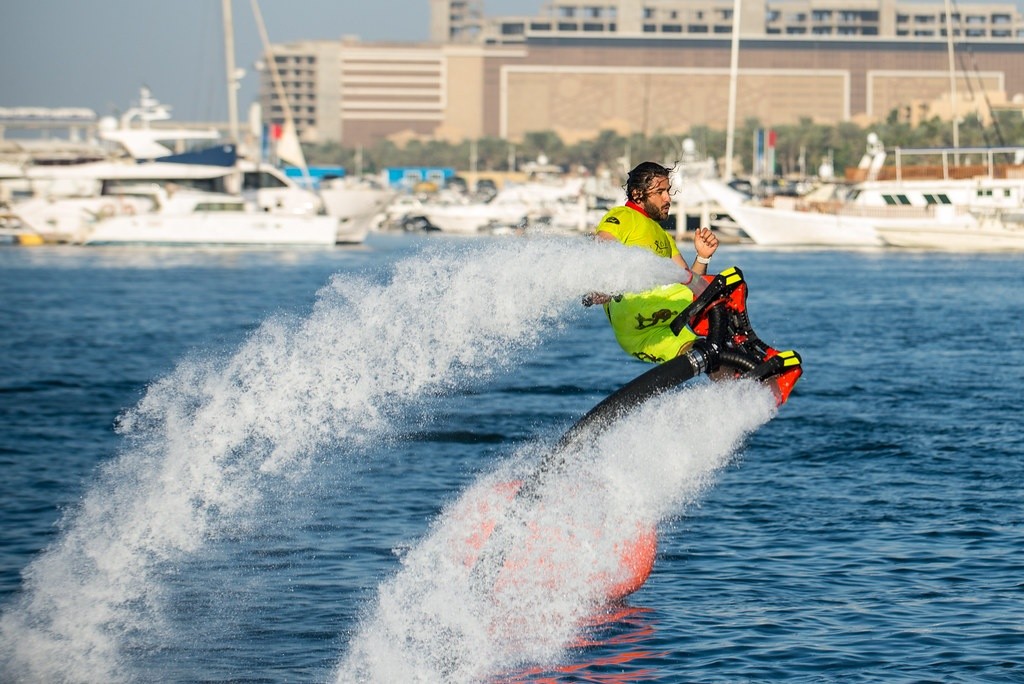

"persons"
[582,161,739,383]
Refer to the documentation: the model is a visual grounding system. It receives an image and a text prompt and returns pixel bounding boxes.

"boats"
[698,147,1024,253]
[0,144,629,247]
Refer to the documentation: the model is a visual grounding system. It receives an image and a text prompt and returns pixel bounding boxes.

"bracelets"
[695,253,713,264]
